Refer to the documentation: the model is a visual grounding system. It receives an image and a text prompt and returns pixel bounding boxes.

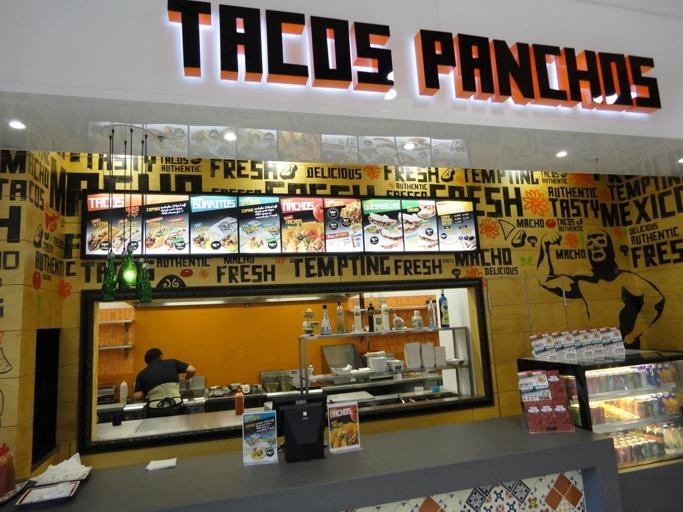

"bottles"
[0,442,16,498]
[643,423,682,458]
[234,389,246,417]
[318,289,449,335]
[119,380,128,404]
[644,364,679,417]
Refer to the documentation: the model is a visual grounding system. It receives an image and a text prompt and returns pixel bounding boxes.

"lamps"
[103,127,154,305]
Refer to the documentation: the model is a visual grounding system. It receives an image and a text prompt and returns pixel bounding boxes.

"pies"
[368,212,439,249]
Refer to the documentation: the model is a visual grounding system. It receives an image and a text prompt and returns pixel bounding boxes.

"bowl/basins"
[368,217,398,229]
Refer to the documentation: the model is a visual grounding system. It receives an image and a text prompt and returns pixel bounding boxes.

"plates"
[403,342,445,370]
[14,479,81,505]
[30,464,93,486]
[249,447,266,459]
[416,211,435,219]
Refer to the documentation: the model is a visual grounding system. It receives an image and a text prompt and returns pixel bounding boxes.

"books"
[144,457,177,471]
[15,453,91,506]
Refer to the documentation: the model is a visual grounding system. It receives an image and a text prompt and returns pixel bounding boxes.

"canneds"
[610,433,658,465]
[571,397,659,426]
[566,370,646,395]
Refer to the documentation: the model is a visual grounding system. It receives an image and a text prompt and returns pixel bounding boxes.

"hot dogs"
[87,227,141,251]
[145,227,171,249]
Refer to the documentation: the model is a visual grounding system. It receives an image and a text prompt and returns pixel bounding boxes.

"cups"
[251,383,260,394]
[230,382,240,392]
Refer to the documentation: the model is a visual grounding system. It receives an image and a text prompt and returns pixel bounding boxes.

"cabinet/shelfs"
[515,347,683,470]
[345,305,434,334]
[296,324,475,401]
[100,319,135,359]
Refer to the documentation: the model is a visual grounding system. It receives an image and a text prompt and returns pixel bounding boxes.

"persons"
[132,347,197,401]
